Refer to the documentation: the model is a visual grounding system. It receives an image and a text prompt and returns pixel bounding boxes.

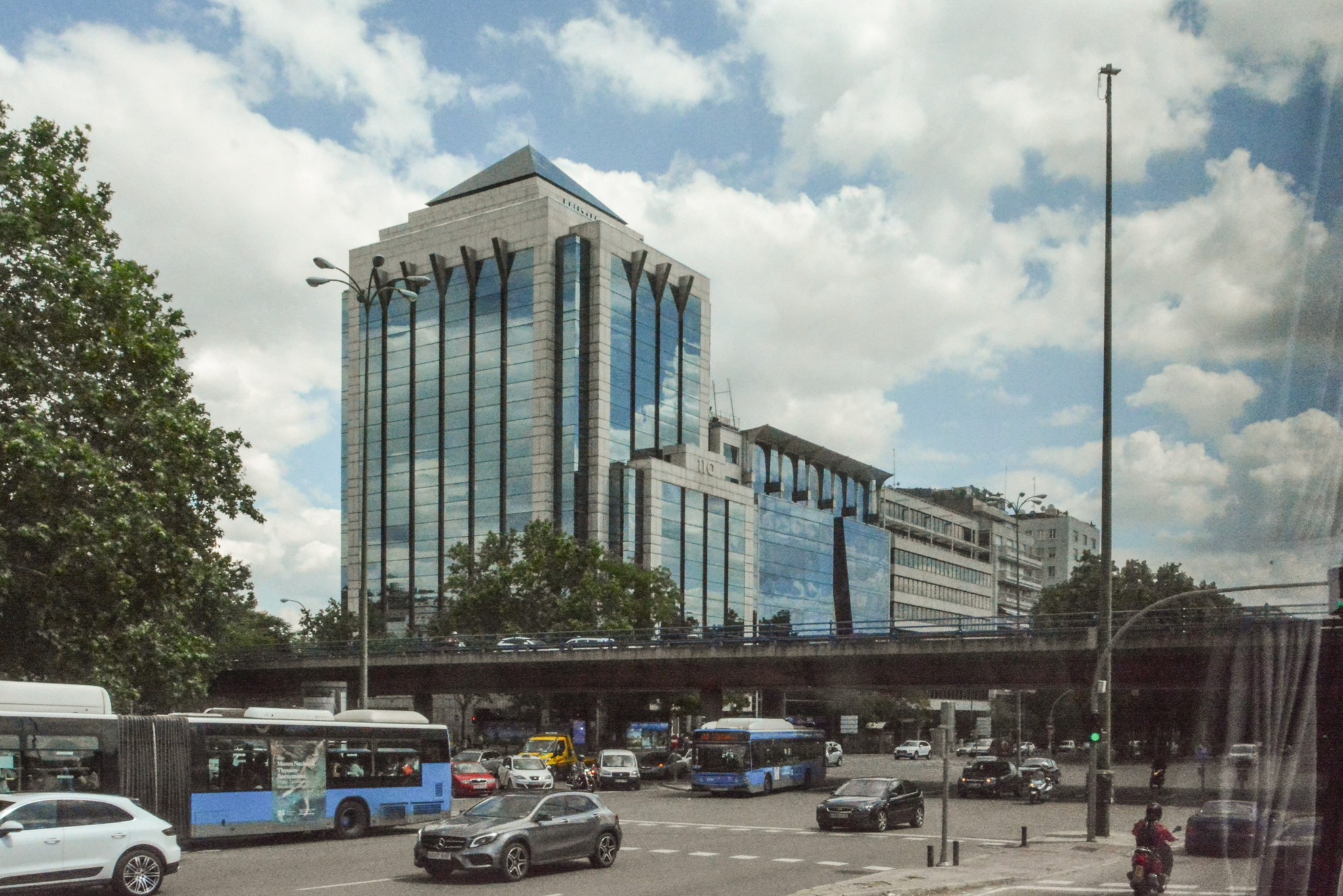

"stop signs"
[670,734,680,747]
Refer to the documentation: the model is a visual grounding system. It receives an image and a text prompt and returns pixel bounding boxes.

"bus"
[1,680,452,845]
[691,718,825,797]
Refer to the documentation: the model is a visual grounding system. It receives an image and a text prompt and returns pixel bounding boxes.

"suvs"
[416,789,623,882]
[0,792,181,896]
[893,740,932,760]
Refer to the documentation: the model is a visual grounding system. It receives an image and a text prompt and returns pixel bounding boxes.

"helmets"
[1145,803,1163,820]
[578,755,585,760]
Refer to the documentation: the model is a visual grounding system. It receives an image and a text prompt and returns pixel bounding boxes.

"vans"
[598,749,641,792]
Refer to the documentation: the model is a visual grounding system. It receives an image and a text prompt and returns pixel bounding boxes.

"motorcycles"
[1028,771,1054,804]
[566,763,597,794]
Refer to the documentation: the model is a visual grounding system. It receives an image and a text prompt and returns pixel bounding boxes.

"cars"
[637,748,699,783]
[1226,744,1259,768]
[449,748,554,799]
[1184,800,1324,873]
[815,777,925,832]
[955,738,1091,799]
[826,741,843,768]
[493,637,615,653]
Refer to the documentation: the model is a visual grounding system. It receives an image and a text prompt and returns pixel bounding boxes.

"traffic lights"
[1090,712,1102,743]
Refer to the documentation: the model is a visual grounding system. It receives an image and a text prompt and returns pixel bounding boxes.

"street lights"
[986,492,1047,629]
[280,598,309,656]
[1091,61,1124,838]
[305,253,433,709]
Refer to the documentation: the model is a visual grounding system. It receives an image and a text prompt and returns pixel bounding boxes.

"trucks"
[517,731,598,782]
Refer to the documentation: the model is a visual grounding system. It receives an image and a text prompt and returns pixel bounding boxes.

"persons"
[1131,802,1177,875]
[571,755,589,786]
[1151,756,1168,778]
[214,753,420,791]
[448,631,459,647]
[1034,762,1051,791]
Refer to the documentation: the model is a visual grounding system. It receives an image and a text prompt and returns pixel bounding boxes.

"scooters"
[1130,825,1181,896]
[1149,769,1166,789]
[443,641,470,655]
